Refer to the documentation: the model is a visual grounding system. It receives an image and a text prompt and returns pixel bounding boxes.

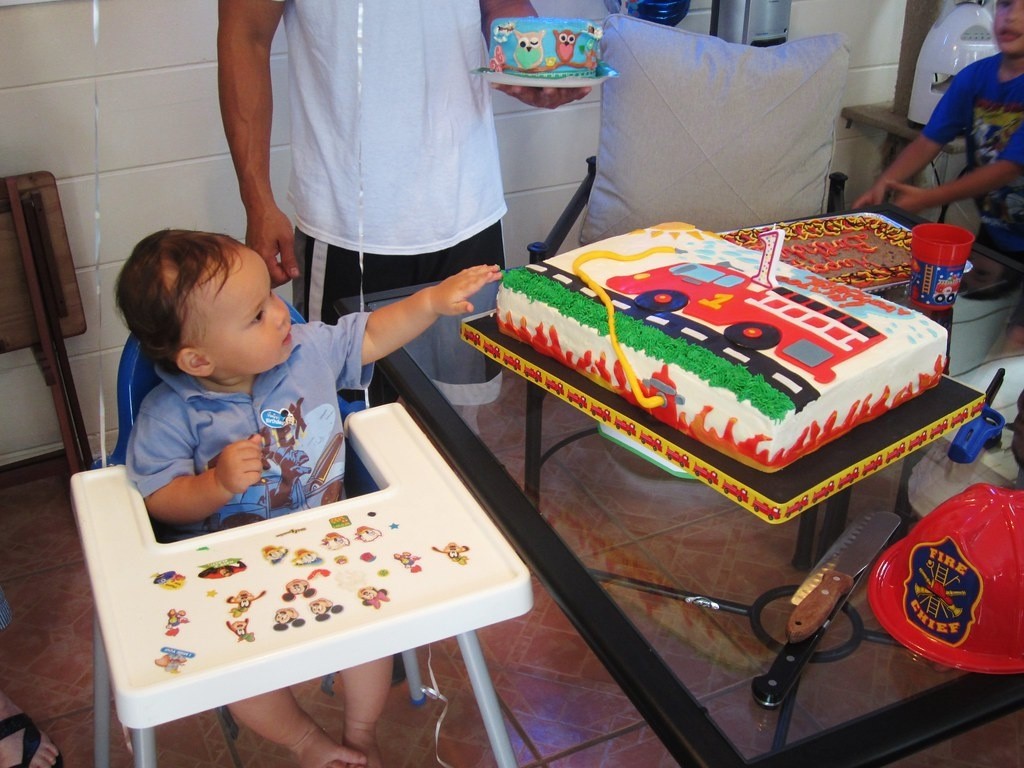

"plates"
[471,61,618,88]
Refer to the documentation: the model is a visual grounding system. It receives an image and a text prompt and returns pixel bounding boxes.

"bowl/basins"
[489,17,603,80]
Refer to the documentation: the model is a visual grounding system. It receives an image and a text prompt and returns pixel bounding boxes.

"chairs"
[86,284,378,768]
[524,155,850,571]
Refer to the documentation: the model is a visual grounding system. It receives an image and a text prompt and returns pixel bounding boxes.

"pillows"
[578,14,850,244]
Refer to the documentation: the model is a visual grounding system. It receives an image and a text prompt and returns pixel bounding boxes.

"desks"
[71,404,535,730]
[844,100,970,223]
[0,171,93,475]
[345,268,1024,768]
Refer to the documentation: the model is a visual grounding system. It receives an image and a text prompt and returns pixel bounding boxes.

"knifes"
[751,567,867,709]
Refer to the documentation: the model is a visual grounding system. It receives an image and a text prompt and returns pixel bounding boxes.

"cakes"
[486,16,604,80]
[497,221,949,473]
[718,216,916,289]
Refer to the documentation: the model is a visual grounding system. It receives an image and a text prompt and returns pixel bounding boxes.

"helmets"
[868,483,1024,675]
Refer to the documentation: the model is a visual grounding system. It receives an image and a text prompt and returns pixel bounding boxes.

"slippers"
[0,708,64,768]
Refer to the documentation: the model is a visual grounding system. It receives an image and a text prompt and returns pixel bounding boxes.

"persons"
[216,0,591,406]
[0,689,63,768]
[848,0,1024,355]
[114,230,501,768]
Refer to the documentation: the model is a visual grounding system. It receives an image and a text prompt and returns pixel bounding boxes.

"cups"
[909,224,975,311]
